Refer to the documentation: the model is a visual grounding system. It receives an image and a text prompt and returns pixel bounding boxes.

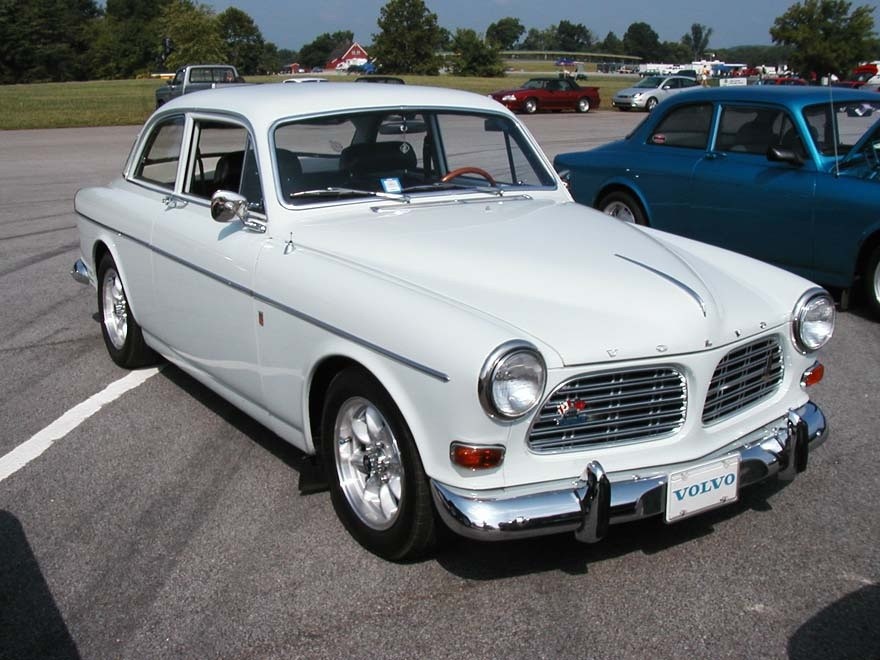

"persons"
[361,54,378,75]
[700,64,710,87]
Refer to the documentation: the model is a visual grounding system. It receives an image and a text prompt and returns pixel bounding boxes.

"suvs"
[671,69,696,77]
[639,69,660,78]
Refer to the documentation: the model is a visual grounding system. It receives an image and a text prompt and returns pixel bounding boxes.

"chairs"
[337,142,417,193]
[204,149,303,206]
[782,125,818,157]
[734,121,773,153]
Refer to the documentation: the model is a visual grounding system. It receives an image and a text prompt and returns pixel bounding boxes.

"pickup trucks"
[154,64,266,124]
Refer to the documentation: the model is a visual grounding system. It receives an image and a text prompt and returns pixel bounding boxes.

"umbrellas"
[553,58,575,73]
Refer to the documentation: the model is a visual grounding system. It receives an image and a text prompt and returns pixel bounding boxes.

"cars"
[730,61,880,92]
[552,84,879,318]
[611,75,705,111]
[354,76,415,121]
[282,79,330,83]
[72,81,836,563]
[488,78,601,115]
